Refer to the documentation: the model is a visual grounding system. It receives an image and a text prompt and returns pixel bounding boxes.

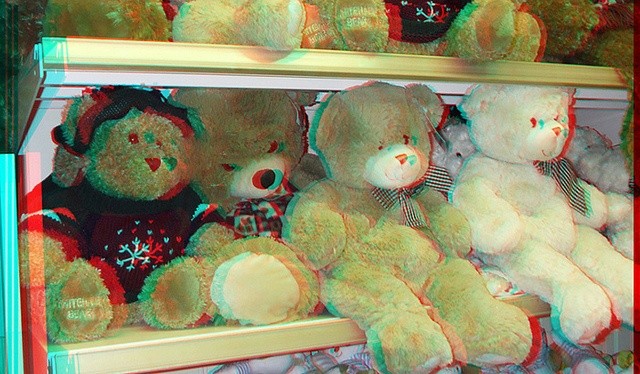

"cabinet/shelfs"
[15,36,634,374]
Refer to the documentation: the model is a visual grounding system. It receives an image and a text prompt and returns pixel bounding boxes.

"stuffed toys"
[282,78,533,374]
[565,125,640,265]
[18,86,210,345]
[447,83,640,345]
[211,328,640,374]
[431,104,510,294]
[43,0,635,65]
[163,89,325,327]
